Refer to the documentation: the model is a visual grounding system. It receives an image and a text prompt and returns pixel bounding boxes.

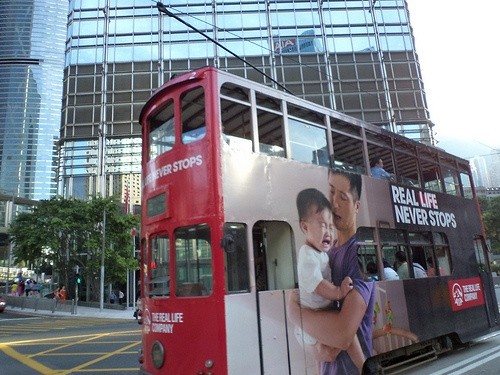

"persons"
[286,161,376,375]
[9,280,67,305]
[296,188,365,375]
[365,261,379,281]
[118,290,124,305]
[110,291,116,304]
[413,262,427,278]
[395,251,409,279]
[383,261,399,281]
[371,157,394,179]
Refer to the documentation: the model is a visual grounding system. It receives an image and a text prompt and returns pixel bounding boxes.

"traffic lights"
[77,277,81,284]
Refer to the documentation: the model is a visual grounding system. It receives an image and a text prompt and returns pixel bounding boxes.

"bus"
[137,65,500,375]
